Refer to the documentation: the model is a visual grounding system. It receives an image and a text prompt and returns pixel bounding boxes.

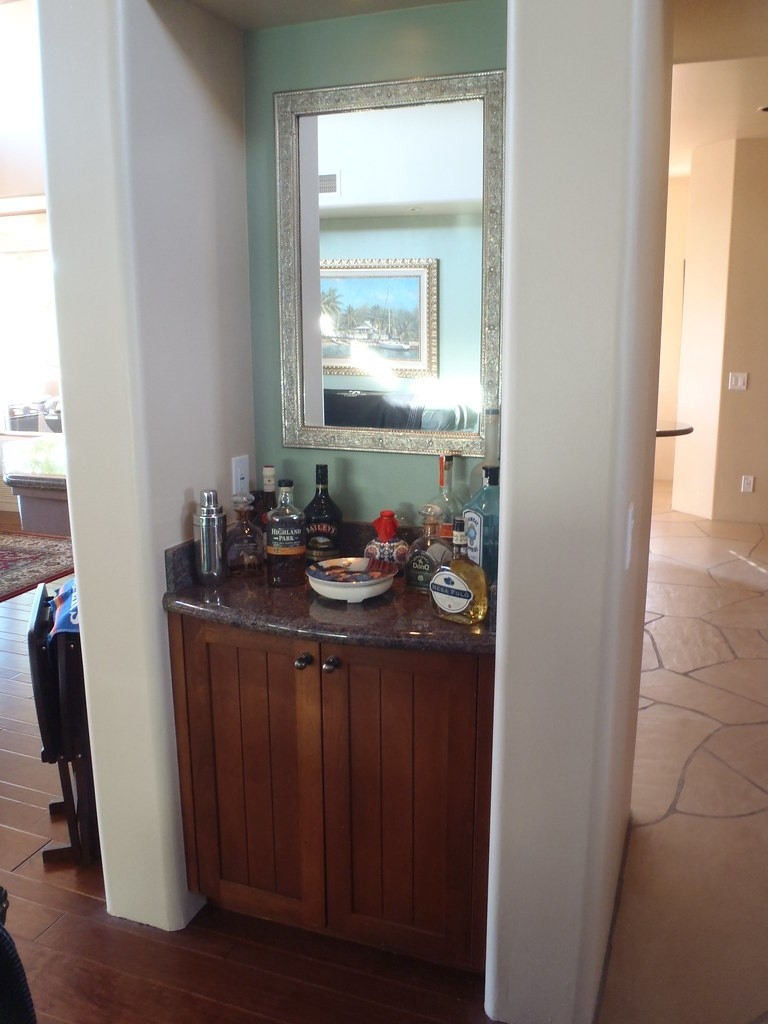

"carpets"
[0,530,74,603]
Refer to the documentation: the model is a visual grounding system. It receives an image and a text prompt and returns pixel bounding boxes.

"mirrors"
[271,68,504,459]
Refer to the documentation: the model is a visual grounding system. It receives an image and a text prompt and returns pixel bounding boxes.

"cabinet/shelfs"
[168,608,496,971]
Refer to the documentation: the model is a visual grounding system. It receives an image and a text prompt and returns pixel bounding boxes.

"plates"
[305,557,398,603]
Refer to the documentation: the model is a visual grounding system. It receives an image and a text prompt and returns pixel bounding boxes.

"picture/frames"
[318,259,440,378]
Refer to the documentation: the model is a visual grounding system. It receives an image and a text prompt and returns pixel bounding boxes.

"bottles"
[428,453,464,551]
[406,505,453,594]
[303,464,341,565]
[265,479,306,586]
[364,510,410,570]
[253,466,279,533]
[462,466,499,585]
[225,493,266,577]
[428,516,489,625]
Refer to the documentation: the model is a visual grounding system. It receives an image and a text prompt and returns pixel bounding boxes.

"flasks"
[193,490,228,587]
